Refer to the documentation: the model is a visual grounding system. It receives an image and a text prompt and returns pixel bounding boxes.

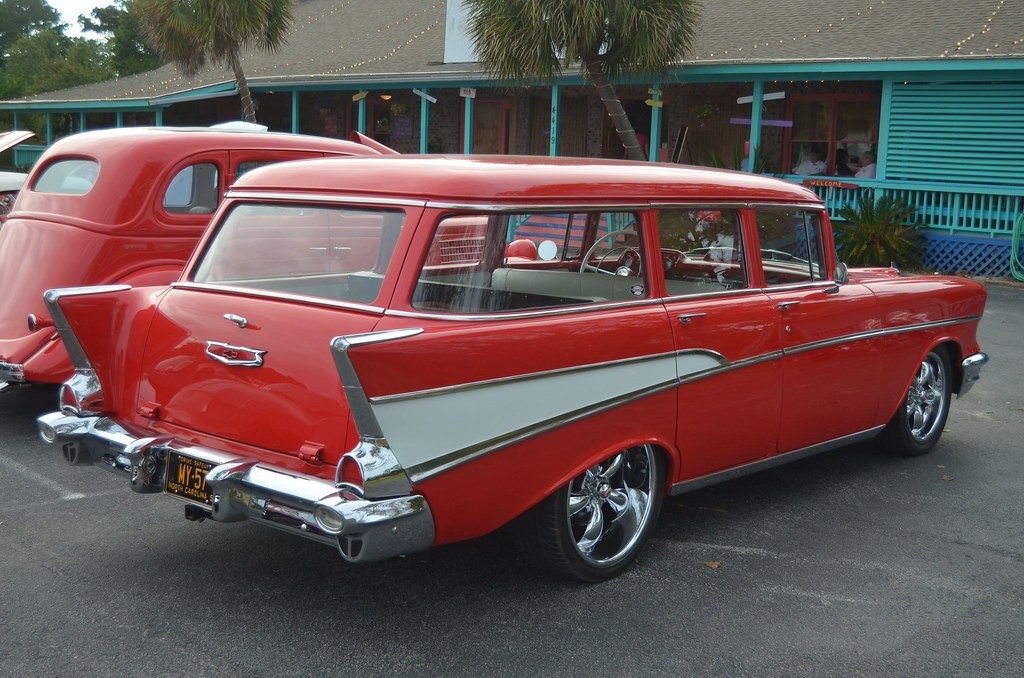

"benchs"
[489,266,728,300]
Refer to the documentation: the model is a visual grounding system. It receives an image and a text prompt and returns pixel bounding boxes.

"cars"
[0,138,54,196]
[0,128,439,413]
[36,151,988,586]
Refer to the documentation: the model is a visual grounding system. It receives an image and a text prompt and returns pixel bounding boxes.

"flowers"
[686,96,722,130]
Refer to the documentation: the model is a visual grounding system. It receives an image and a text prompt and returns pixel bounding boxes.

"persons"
[854,151,876,178]
[634,131,648,158]
[835,148,853,176]
[798,148,828,175]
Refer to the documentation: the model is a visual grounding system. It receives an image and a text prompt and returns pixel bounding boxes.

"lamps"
[380,93,394,101]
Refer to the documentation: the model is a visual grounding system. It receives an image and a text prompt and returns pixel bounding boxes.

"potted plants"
[390,102,412,116]
